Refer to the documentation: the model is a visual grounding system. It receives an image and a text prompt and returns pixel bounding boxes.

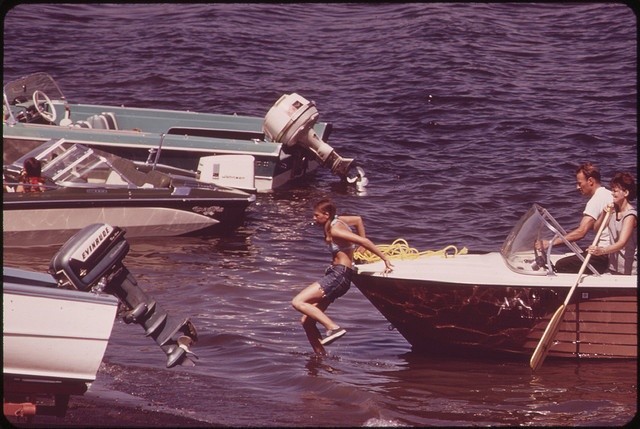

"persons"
[6,157,48,193]
[535,163,615,274]
[291,199,396,355]
[586,171,638,275]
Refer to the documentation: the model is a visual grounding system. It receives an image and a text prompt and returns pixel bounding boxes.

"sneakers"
[321,327,347,346]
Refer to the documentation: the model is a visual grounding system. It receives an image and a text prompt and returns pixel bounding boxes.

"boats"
[351,203,640,358]
[3,72,369,196]
[3,135,257,247]
[3,222,199,428]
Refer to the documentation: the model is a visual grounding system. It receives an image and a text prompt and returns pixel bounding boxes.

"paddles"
[531,203,613,373]
